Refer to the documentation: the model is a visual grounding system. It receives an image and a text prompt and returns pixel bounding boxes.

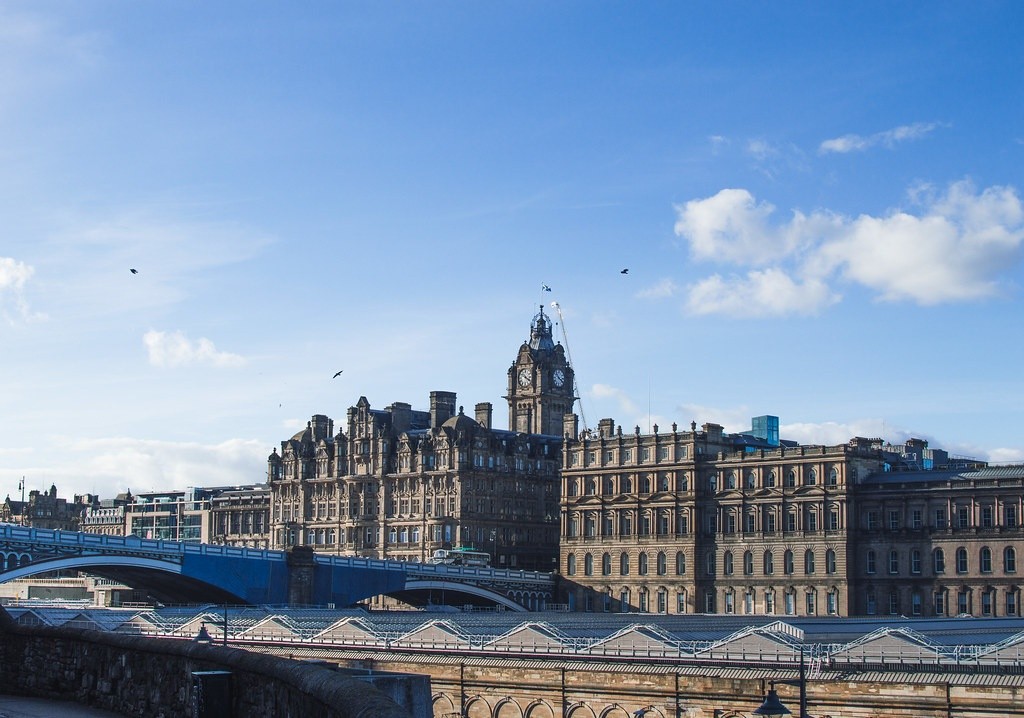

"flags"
[542,284,551,292]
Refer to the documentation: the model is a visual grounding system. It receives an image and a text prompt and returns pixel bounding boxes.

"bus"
[433,542,490,567]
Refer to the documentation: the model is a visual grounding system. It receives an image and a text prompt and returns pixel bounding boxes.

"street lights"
[750,648,811,718]
[191,604,228,646]
[18,476,24,528]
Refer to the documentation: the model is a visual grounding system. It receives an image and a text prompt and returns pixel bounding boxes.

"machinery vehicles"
[551,301,592,440]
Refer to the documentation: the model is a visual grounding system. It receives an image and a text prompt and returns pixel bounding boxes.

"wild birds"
[332,371,343,378]
[621,268,629,274]
[129,268,139,275]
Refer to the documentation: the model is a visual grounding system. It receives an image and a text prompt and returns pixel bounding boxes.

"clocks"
[518,368,532,388]
[552,368,565,388]
